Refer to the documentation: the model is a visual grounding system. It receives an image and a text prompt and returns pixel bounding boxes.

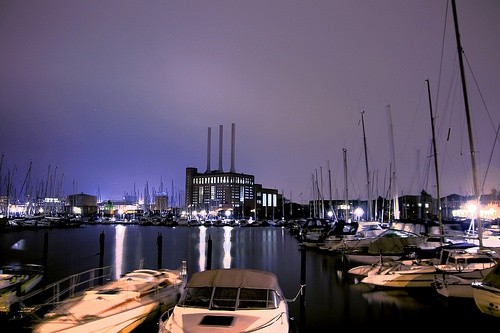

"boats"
[0,227,188,333]
[0,231,52,314]
[152,233,309,333]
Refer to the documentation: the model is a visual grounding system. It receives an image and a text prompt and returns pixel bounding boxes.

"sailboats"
[285,1,500,320]
[0,159,295,228]
